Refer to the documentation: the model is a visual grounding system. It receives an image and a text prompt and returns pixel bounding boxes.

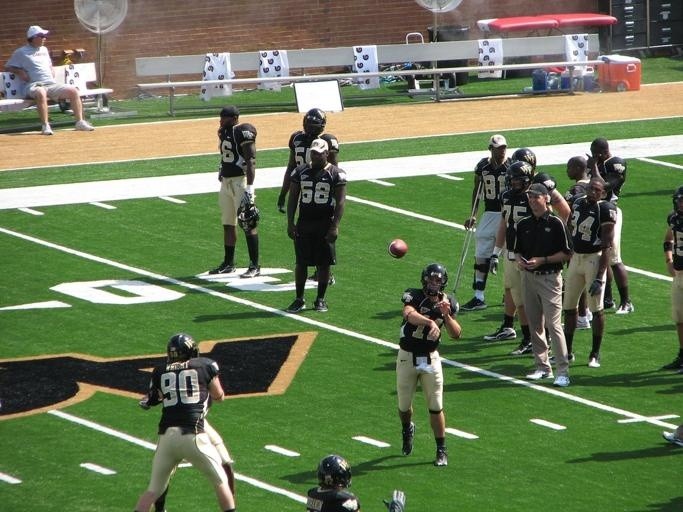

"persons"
[275,108,341,287]
[660,421,681,450]
[394,263,463,468]
[148,393,237,512]
[461,135,636,387]
[283,138,348,314]
[660,186,683,370]
[2,24,94,136]
[304,454,407,511]
[207,104,261,279]
[133,332,236,512]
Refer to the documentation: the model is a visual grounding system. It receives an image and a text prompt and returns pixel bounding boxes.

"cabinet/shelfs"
[598,0,683,58]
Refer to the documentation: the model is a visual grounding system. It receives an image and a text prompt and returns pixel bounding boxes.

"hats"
[488,134,507,149]
[523,182,548,195]
[311,139,329,154]
[27,25,50,38]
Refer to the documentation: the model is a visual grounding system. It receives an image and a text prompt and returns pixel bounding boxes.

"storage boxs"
[598,55,641,91]
[560,66,595,91]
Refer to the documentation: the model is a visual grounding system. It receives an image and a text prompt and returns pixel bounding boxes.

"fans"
[72,0,128,114]
[413,0,462,102]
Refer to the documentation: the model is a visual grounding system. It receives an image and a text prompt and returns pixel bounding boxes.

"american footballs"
[387,239,408,258]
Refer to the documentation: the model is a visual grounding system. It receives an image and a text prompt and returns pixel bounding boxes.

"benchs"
[134,33,606,118]
[0,63,114,114]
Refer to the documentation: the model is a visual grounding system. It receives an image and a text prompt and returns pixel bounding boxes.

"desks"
[476,13,618,38]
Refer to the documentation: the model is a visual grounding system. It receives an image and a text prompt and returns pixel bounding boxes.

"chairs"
[406,32,458,93]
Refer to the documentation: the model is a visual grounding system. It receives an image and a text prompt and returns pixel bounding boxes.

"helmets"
[237,205,260,232]
[302,108,326,134]
[672,186,683,214]
[421,263,449,292]
[318,454,352,488]
[512,148,537,167]
[505,160,534,185]
[166,333,198,360]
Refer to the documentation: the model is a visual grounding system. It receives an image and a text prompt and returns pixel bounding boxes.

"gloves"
[489,255,500,274]
[239,191,254,207]
[383,490,406,511]
[588,279,603,297]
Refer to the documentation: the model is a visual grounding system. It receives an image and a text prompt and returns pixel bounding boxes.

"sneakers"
[314,298,328,312]
[548,353,575,364]
[435,448,448,466]
[41,120,54,135]
[308,271,336,285]
[401,422,415,455]
[662,355,682,371]
[509,341,552,355]
[662,427,682,448]
[526,370,553,380]
[461,296,487,310]
[240,266,260,278]
[561,301,634,328]
[554,376,571,387]
[207,262,236,274]
[75,120,95,132]
[484,327,517,341]
[588,358,601,368]
[286,299,306,313]
